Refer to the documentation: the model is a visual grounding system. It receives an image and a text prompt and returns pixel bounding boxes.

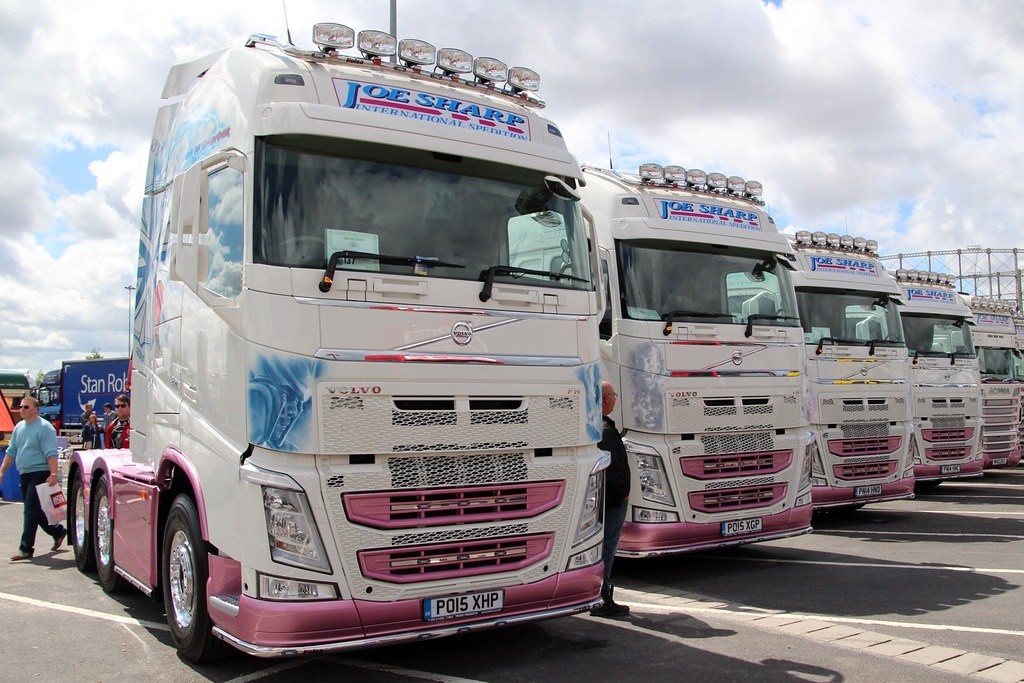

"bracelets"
[51,473,57,475]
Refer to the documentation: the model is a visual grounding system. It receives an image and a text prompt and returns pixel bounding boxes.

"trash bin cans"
[0,440,19,501]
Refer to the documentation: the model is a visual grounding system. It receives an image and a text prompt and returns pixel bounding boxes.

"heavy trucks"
[932,292,1024,469]
[32,356,131,449]
[509,162,814,558]
[67,22,611,664]
[726,231,915,515]
[845,269,984,487]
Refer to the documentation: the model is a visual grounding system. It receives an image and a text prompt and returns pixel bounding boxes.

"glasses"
[18,405,35,409]
[115,404,129,408]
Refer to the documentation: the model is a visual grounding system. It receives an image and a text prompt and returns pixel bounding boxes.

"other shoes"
[11,551,33,561]
[592,602,630,618]
[52,528,68,551]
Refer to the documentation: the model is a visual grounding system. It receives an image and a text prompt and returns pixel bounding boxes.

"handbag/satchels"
[84,441,93,450]
[36,482,68,526]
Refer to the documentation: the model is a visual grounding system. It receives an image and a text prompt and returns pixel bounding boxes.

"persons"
[0,397,67,560]
[105,395,130,449]
[82,404,92,448]
[589,381,631,616]
[633,343,663,429]
[82,414,104,449]
[103,403,117,426]
